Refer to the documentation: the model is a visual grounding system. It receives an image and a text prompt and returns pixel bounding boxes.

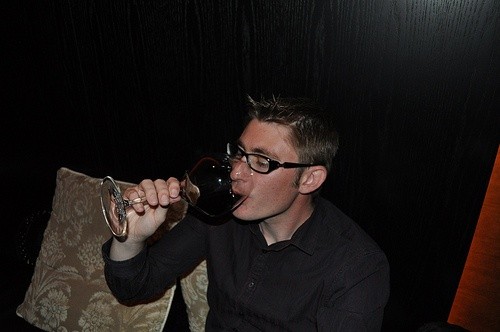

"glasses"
[226,140,320,174]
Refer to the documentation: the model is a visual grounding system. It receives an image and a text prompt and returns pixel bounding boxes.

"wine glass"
[100,149,252,238]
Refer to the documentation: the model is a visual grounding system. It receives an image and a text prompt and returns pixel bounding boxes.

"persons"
[99,90,393,332]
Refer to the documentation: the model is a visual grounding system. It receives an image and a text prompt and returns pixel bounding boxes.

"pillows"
[17,168,187,332]
[181,260,209,332]
